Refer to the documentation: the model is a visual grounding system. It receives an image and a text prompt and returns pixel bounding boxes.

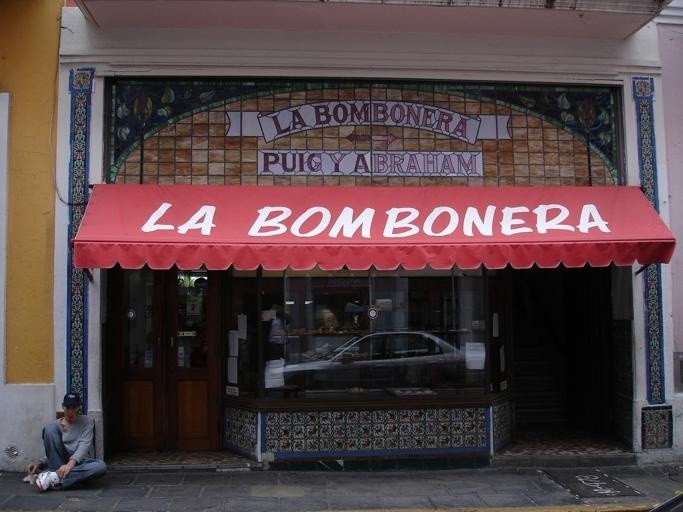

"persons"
[23,393,108,492]
[270,311,289,358]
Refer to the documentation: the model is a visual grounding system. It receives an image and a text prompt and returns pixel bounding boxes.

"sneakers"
[36,472,59,490]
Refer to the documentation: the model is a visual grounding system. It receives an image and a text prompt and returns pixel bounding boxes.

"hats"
[62,393,80,407]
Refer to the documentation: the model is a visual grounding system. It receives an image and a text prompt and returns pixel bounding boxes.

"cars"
[258,329,465,391]
